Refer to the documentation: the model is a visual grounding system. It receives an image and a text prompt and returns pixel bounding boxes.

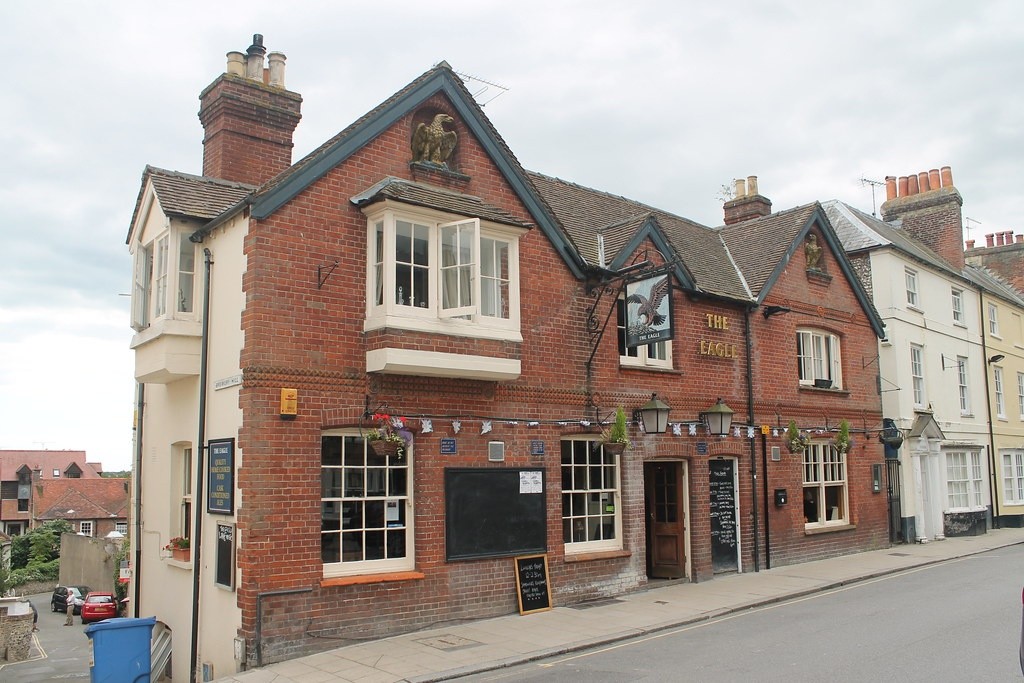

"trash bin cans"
[84,616,156,683]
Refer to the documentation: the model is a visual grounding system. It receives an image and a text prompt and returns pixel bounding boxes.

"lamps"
[988,354,1004,365]
[699,397,734,435]
[868,428,903,450]
[632,392,671,434]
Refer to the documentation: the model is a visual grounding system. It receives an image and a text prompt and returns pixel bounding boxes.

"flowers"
[361,412,406,458]
[785,415,809,451]
[163,537,189,551]
[591,404,637,452]
[828,418,858,452]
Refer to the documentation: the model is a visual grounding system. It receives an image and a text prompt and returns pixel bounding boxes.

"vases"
[171,547,190,561]
[789,445,805,454]
[372,440,399,456]
[834,443,851,453]
[603,442,626,454]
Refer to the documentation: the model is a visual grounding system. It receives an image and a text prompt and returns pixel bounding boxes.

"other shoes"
[63,623,73,627]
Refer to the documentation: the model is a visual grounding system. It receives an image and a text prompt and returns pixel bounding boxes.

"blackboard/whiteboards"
[709,456,742,574]
[213,520,236,592]
[443,466,547,562]
[515,554,552,616]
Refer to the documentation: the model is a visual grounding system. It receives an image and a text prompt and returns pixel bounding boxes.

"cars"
[51,585,93,615]
[81,592,119,624]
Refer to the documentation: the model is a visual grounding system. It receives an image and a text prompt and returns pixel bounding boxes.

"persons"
[64,589,76,626]
